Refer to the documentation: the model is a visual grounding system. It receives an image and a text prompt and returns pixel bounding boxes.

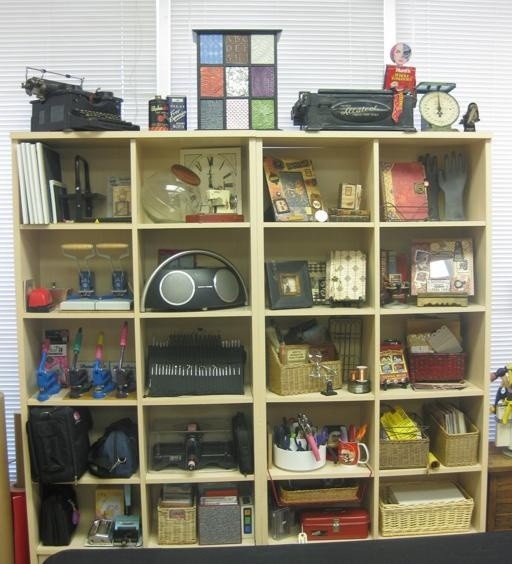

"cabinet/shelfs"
[11,130,492,564]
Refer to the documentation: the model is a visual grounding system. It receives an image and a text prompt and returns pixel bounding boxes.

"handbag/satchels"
[89,419,139,479]
[39,486,78,545]
[26,406,91,484]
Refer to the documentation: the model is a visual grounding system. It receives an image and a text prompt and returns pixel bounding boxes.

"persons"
[384,41,418,124]
[418,151,446,220]
[437,148,469,220]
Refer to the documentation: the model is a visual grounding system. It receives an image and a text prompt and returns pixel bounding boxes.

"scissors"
[339,422,368,442]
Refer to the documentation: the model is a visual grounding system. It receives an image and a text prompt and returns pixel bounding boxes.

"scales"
[415,81,460,132]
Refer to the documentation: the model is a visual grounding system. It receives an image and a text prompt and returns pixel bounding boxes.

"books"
[160,483,239,509]
[385,479,467,504]
[15,140,70,226]
[423,397,468,435]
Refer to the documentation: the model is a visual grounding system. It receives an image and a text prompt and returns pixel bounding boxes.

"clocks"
[419,92,460,131]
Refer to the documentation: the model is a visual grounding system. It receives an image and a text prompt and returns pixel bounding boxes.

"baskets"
[267,342,341,397]
[279,481,359,504]
[380,481,475,537]
[380,410,432,470]
[156,500,198,545]
[426,410,479,465]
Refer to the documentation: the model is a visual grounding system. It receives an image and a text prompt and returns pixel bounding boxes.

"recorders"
[141,249,249,313]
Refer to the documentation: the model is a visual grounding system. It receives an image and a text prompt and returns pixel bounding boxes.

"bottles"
[140,162,204,224]
